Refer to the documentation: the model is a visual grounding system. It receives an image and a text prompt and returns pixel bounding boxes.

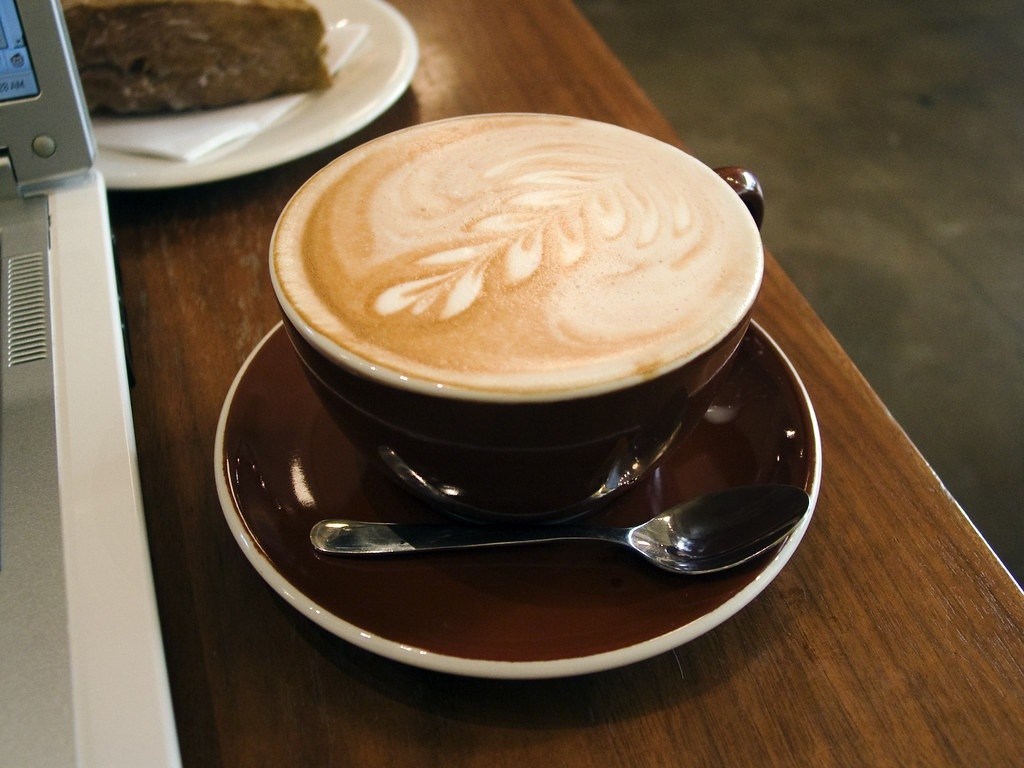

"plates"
[212,316,822,680]
[90,0,419,188]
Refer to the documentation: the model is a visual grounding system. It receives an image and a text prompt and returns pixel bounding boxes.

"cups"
[268,113,765,535]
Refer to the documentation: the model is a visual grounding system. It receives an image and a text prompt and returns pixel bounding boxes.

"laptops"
[0,1,182,767]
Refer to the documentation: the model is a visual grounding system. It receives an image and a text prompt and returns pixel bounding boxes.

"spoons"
[309,487,809,574]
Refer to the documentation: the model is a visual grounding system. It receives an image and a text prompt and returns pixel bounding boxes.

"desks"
[103,1,1024,768]
